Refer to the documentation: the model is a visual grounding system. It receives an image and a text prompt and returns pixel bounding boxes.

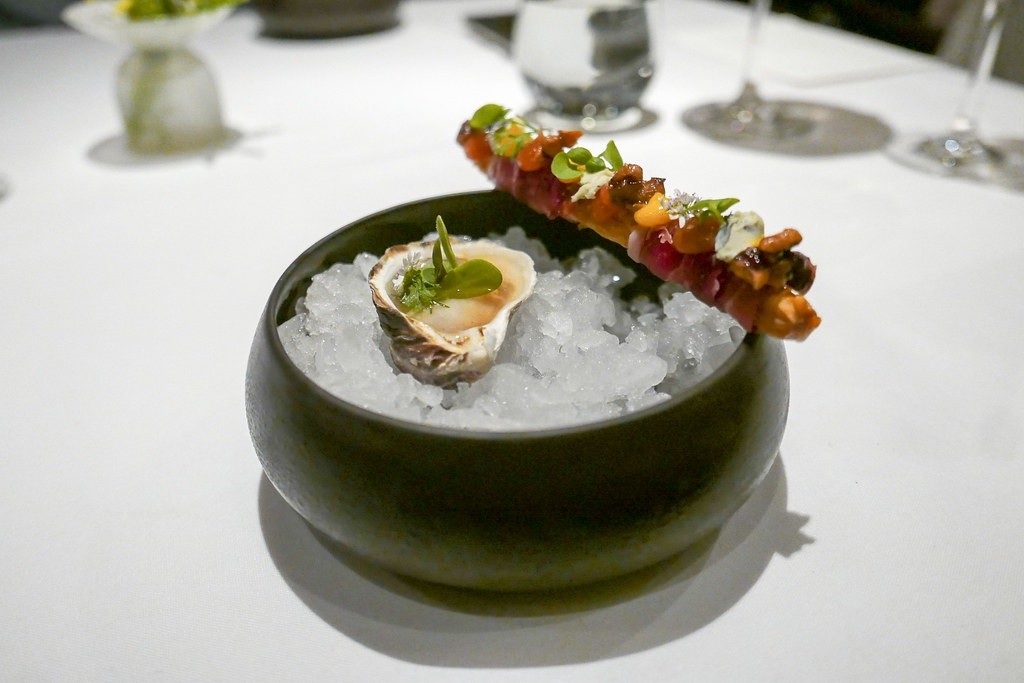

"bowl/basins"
[249,0,401,39]
[246,188,791,600]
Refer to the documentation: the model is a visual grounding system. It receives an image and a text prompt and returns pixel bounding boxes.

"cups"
[512,0,654,133]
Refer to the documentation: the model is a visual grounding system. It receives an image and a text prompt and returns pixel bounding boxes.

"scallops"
[368,235,538,385]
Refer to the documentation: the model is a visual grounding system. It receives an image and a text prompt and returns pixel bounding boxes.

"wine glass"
[710,0,819,141]
[919,0,1011,166]
